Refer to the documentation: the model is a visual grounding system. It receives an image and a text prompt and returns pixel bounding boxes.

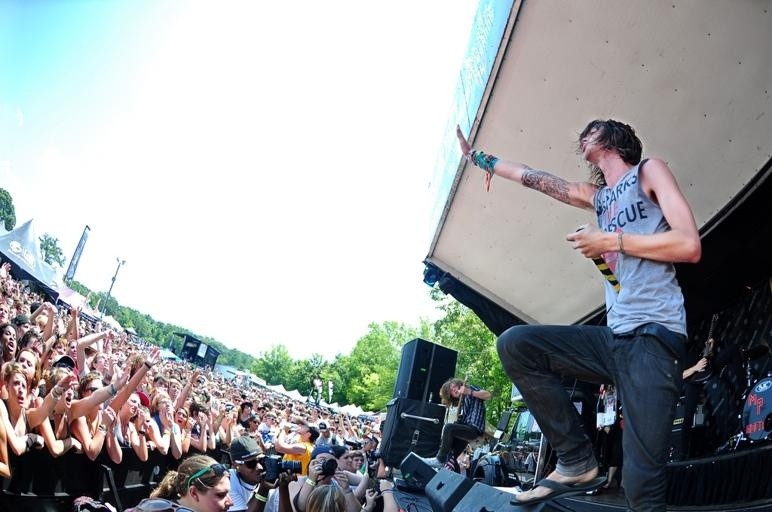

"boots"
[362,435,379,445]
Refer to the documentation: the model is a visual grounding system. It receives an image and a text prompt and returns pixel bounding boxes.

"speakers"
[399,451,437,492]
[379,399,446,468]
[424,467,474,512]
[450,481,575,512]
[393,338,459,405]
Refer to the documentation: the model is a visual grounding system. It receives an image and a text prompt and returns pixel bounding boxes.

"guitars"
[453,376,467,423]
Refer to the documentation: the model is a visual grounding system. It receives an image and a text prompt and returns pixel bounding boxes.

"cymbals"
[741,343,768,361]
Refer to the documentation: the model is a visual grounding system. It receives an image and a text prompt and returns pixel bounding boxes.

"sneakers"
[422,457,445,468]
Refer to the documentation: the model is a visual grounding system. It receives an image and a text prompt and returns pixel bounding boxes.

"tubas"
[692,313,720,382]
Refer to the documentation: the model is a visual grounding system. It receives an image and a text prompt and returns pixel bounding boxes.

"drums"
[740,376,772,444]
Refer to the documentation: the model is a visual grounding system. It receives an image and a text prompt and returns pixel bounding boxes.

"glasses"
[234,456,268,470]
[58,361,74,372]
[187,463,231,493]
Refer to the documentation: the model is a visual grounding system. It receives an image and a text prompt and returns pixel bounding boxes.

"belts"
[614,322,684,358]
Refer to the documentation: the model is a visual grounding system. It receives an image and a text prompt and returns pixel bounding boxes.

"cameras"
[318,458,337,477]
[265,454,302,482]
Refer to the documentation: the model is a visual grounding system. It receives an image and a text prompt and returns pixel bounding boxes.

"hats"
[317,422,327,433]
[230,435,263,460]
[136,391,150,408]
[51,355,75,368]
[305,444,338,471]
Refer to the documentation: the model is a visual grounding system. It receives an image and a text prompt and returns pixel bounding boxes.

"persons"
[454,118,703,511]
[1,262,398,512]
[595,382,615,412]
[600,404,624,491]
[676,332,729,459]
[422,377,530,479]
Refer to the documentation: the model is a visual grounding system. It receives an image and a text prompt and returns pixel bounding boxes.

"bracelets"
[466,147,500,174]
[617,230,625,255]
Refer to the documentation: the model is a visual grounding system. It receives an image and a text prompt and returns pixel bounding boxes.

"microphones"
[575,228,621,295]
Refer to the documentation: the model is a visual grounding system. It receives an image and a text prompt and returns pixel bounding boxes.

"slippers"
[510,476,608,506]
